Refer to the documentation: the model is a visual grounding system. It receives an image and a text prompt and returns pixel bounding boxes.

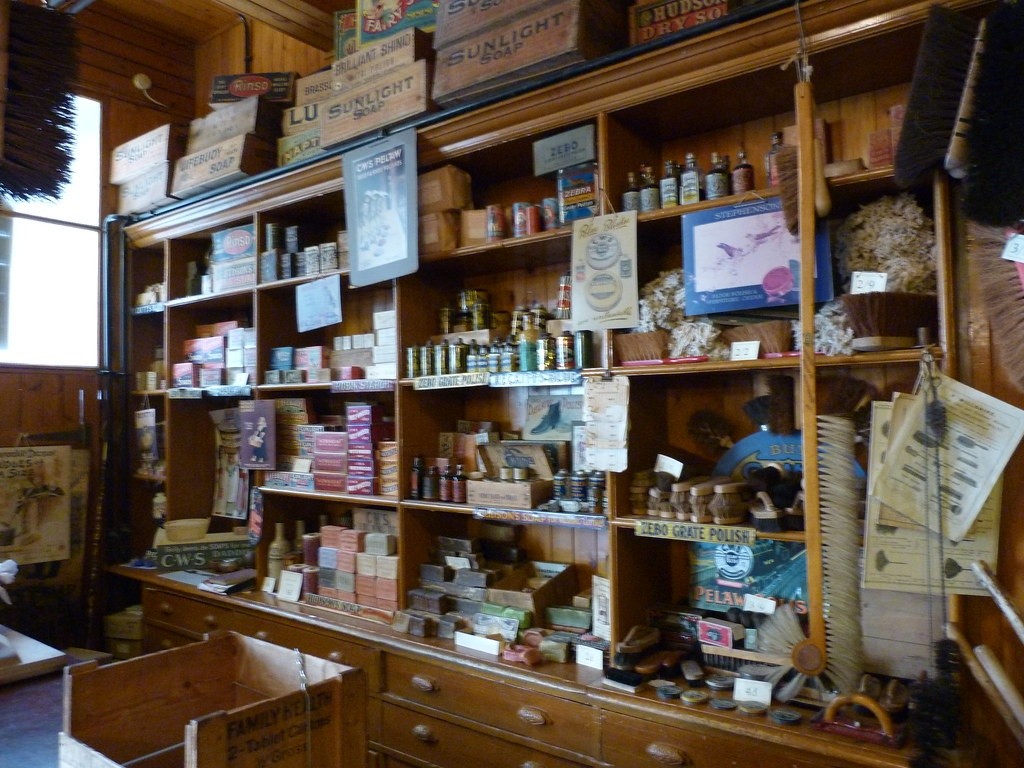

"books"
[199,569,256,595]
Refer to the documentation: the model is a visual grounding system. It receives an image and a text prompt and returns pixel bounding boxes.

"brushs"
[601,668,644,694]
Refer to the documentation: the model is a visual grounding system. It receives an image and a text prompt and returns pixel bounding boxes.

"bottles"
[405,329,575,377]
[452,464,468,503]
[553,469,605,513]
[411,457,422,498]
[439,464,451,502]
[680,152,703,204]
[732,152,754,194]
[422,466,439,500]
[621,168,639,215]
[765,132,783,186]
[706,152,729,201]
[639,164,659,210]
[659,159,679,209]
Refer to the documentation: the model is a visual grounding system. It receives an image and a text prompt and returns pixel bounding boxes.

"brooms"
[963,218,1024,386]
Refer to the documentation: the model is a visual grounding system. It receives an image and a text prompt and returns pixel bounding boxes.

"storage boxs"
[110,0,738,299]
[171,321,258,385]
[258,311,398,497]
[440,432,566,509]
[58,606,367,768]
[487,561,580,627]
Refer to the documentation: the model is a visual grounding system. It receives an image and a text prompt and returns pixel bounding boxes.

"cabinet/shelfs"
[86,0,1024,768]
